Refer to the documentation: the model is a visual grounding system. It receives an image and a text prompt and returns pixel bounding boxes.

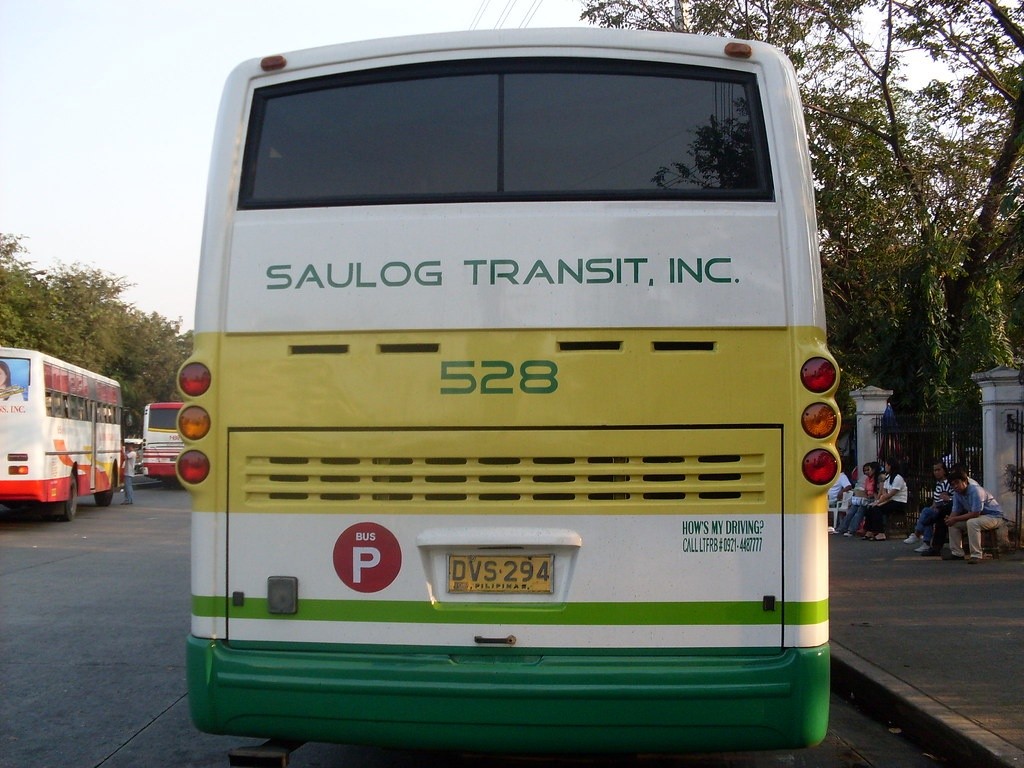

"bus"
[175,26,846,768]
[0,345,125,524]
[141,403,188,483]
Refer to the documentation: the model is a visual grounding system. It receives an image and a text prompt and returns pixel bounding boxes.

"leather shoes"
[861,534,886,540]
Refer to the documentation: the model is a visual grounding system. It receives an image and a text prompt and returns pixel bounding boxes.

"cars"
[124,438,143,474]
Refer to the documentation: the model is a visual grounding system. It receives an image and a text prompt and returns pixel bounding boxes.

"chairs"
[828,488,854,530]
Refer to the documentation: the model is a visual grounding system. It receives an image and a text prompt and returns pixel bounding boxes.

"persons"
[921,461,978,557]
[943,472,1004,565]
[828,462,908,542]
[121,442,135,504]
[903,462,951,551]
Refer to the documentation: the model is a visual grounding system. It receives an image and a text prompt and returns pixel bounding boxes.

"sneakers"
[903,533,920,543]
[828,525,836,534]
[843,530,851,536]
[967,555,982,563]
[913,542,931,552]
[921,546,939,557]
[942,551,962,560]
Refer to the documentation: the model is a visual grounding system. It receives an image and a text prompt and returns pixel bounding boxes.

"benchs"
[978,528,1002,560]
